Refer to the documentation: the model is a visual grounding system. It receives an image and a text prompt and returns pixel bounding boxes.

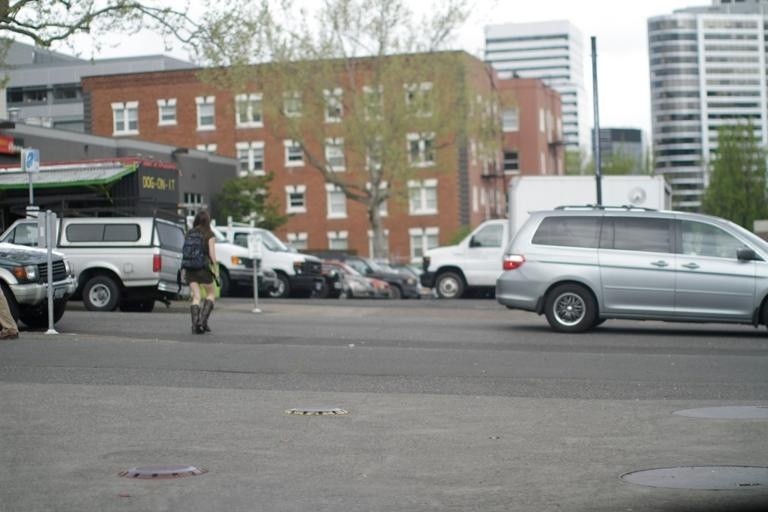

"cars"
[211,216,419,300]
[0,207,191,328]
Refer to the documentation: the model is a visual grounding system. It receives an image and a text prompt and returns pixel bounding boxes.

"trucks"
[420,174,673,299]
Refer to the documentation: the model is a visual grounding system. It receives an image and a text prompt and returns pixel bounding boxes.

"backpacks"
[180,227,208,270]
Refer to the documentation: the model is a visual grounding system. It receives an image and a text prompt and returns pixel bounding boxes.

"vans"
[495,204,768,333]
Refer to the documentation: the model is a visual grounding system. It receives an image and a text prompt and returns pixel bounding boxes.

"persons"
[0,288,18,340]
[181,210,221,335]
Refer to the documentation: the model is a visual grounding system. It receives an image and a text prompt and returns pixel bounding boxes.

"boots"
[190,305,205,335]
[197,298,214,332]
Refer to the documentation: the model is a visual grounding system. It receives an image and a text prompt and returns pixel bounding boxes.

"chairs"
[553,222,717,256]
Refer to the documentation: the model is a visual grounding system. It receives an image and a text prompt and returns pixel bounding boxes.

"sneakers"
[1,327,19,339]
